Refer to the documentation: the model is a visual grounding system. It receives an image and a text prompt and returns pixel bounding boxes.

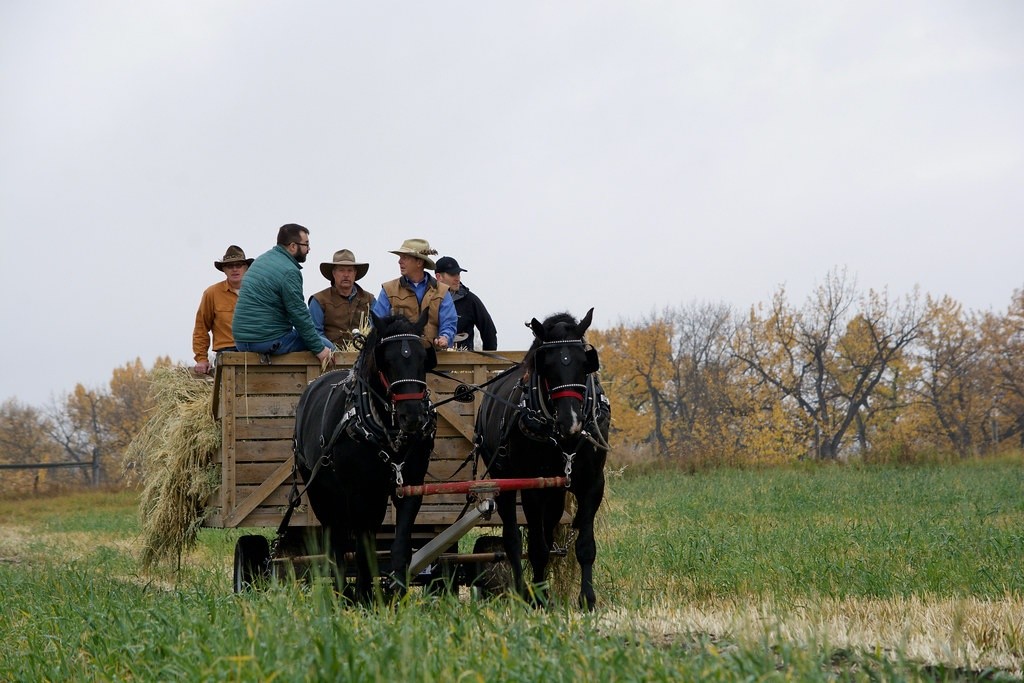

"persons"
[373,239,458,349]
[232,224,335,368]
[309,249,377,352]
[193,245,254,375]
[435,256,498,352]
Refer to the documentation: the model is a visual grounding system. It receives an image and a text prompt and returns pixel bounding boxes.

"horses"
[475,308,611,612]
[293,306,436,609]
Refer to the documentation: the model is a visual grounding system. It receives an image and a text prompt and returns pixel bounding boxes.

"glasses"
[289,241,309,248]
[223,263,246,269]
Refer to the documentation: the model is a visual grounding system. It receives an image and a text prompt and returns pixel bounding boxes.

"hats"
[214,245,254,272]
[388,239,437,270]
[320,249,369,281]
[434,256,468,275]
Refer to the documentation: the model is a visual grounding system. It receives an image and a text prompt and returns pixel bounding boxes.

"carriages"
[199,306,613,614]
